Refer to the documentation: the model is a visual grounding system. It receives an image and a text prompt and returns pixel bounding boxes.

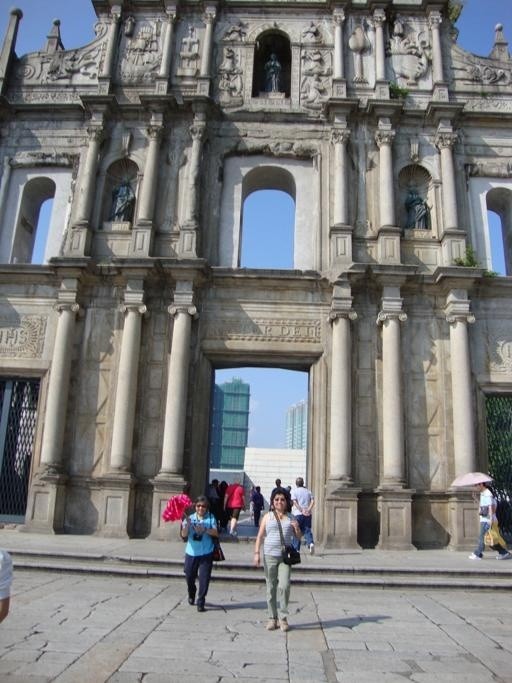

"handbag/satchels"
[282,544,300,565]
[484,528,499,546]
[480,506,489,516]
[213,547,225,561]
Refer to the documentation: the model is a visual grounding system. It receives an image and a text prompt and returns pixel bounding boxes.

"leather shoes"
[189,592,195,604]
[198,604,204,612]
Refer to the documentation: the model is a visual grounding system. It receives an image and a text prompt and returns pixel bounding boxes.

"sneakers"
[267,616,290,632]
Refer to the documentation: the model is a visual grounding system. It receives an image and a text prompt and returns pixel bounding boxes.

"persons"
[484,485,508,556]
[218,73,235,97]
[465,480,509,560]
[109,174,135,220]
[304,72,325,101]
[180,476,316,636]
[403,178,432,230]
[263,49,282,90]
[0,550,13,626]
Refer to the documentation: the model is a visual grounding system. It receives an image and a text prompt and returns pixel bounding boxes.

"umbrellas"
[161,492,192,524]
[450,471,495,488]
